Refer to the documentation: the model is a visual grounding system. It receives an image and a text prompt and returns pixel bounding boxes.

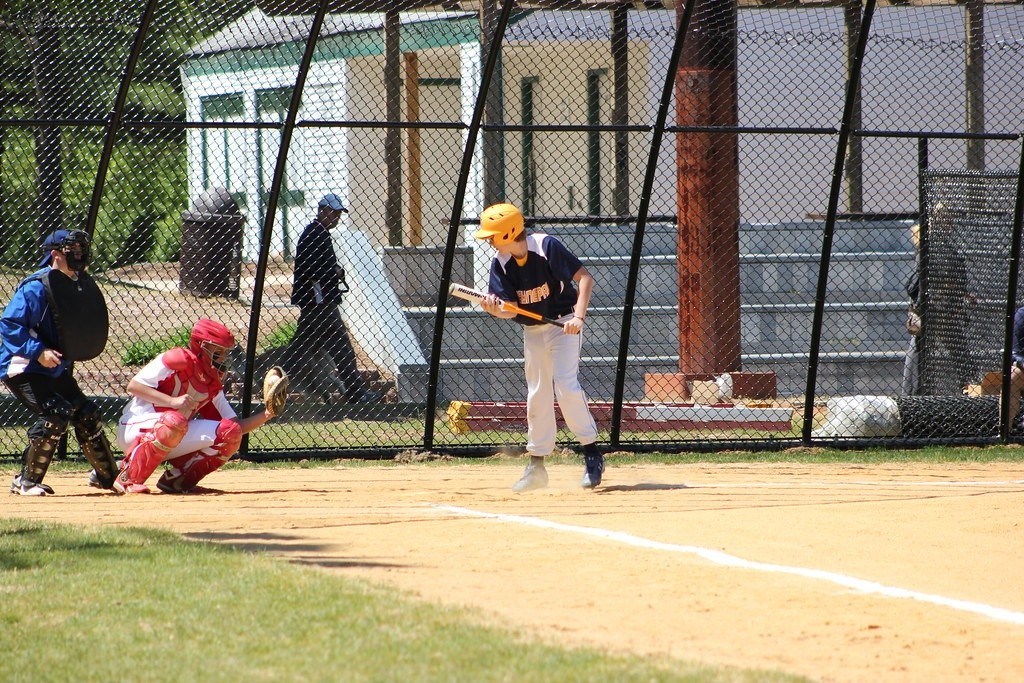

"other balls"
[300,459,310,468]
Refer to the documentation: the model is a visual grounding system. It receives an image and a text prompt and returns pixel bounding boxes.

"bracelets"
[573,316,584,322]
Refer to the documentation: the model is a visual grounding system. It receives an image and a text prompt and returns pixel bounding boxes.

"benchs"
[376,219,1024,402]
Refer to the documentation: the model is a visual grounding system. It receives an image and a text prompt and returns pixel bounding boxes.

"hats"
[318,193,348,213]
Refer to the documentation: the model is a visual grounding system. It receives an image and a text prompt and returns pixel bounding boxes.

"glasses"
[484,238,494,245]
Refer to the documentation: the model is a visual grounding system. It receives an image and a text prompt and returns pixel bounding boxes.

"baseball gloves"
[263,366,289,416]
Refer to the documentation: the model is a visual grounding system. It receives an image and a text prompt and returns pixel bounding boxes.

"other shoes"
[349,391,383,404]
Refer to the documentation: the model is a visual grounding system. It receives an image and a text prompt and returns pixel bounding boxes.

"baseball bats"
[449,283,580,334]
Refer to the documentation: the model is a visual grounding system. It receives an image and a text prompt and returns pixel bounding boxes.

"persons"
[901,204,981,400]
[474,204,606,493]
[0,228,121,495]
[250,193,383,407]
[1009,307,1024,436]
[110,318,289,494]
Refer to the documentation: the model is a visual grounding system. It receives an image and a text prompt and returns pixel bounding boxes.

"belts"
[524,306,575,326]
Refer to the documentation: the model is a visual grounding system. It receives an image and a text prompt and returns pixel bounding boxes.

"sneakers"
[10,473,46,496]
[87,469,112,490]
[113,467,151,494]
[512,465,549,493]
[156,471,194,495]
[580,452,605,489]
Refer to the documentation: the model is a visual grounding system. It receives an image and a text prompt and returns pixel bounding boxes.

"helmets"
[473,204,525,247]
[190,319,235,380]
[38,229,72,269]
